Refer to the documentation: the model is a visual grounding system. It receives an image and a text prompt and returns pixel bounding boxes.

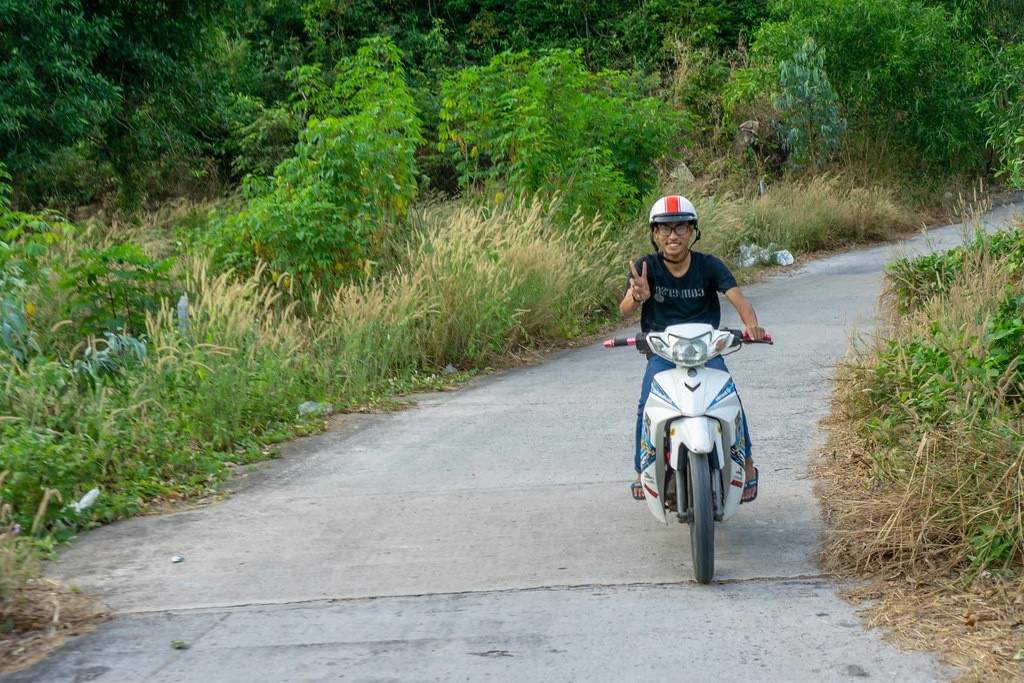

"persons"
[619,195,766,504]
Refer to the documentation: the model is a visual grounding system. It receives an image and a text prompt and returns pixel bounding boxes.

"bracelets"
[632,295,643,303]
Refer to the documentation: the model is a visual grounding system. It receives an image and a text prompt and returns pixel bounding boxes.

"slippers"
[741,467,758,502]
[631,481,646,501]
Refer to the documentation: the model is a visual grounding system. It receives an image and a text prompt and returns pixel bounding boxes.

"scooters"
[604,323,774,584]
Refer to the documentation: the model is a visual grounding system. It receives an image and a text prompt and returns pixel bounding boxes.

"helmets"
[648,195,698,228]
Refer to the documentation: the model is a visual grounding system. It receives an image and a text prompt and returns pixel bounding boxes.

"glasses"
[652,223,693,237]
